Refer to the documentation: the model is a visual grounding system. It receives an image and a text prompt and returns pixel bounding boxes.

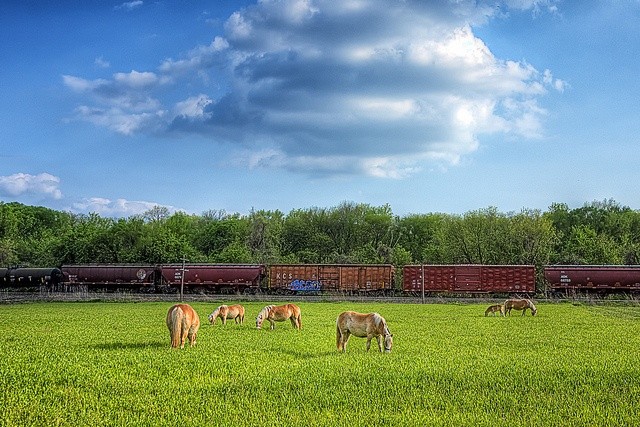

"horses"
[255,302,302,330]
[504,298,537,317]
[335,310,393,353]
[208,303,245,324]
[167,304,200,348]
[484,304,505,316]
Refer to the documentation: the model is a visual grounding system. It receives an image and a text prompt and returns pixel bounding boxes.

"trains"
[0,263,640,299]
